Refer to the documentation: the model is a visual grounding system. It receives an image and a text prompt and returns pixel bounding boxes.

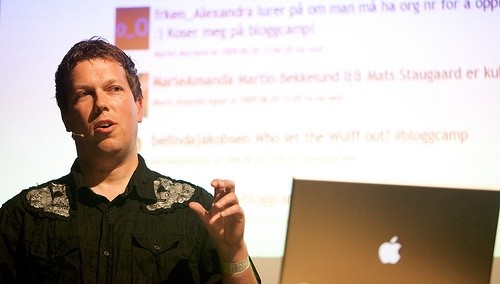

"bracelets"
[220,255,251,275]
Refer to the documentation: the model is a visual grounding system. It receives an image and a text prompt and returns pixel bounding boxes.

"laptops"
[279,179,500,284]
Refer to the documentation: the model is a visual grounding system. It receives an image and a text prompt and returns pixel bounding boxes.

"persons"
[0,36,261,284]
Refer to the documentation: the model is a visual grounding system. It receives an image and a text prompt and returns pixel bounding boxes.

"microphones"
[72,132,84,138]
